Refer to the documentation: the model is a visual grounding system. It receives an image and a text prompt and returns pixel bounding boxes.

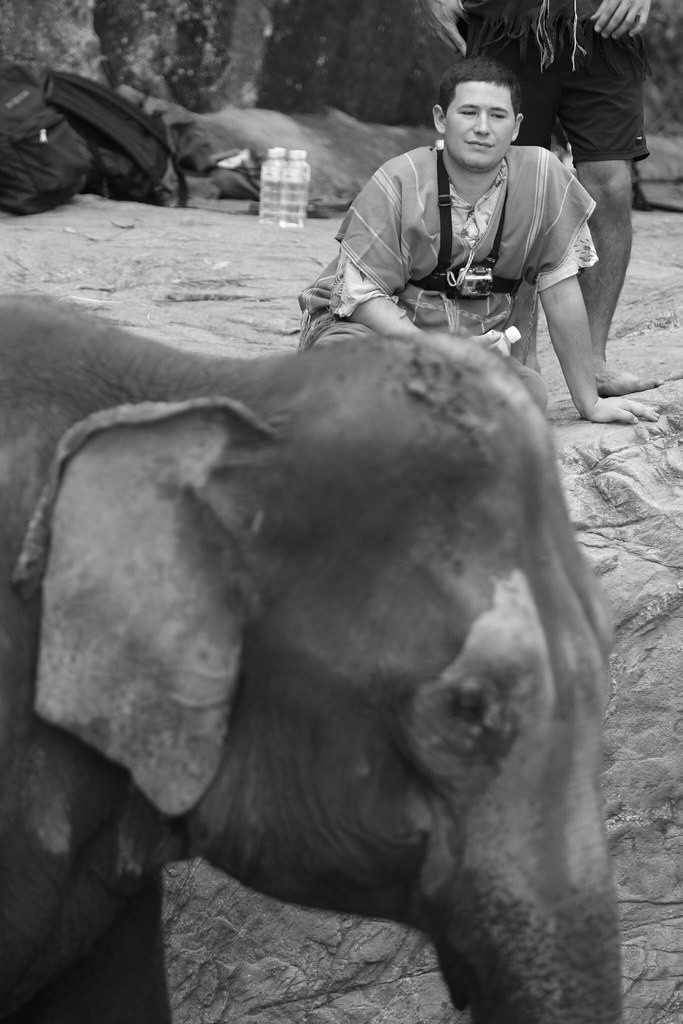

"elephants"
[0,291,621,1024]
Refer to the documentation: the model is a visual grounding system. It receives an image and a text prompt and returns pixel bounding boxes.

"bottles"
[258,145,312,230]
[478,325,522,358]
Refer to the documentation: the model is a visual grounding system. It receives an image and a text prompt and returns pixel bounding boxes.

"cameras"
[456,268,494,296]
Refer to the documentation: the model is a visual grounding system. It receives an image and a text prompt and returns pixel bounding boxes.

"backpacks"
[0,65,262,215]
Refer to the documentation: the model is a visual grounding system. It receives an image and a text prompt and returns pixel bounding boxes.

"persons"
[300,58,661,424]
[417,1,664,397]
